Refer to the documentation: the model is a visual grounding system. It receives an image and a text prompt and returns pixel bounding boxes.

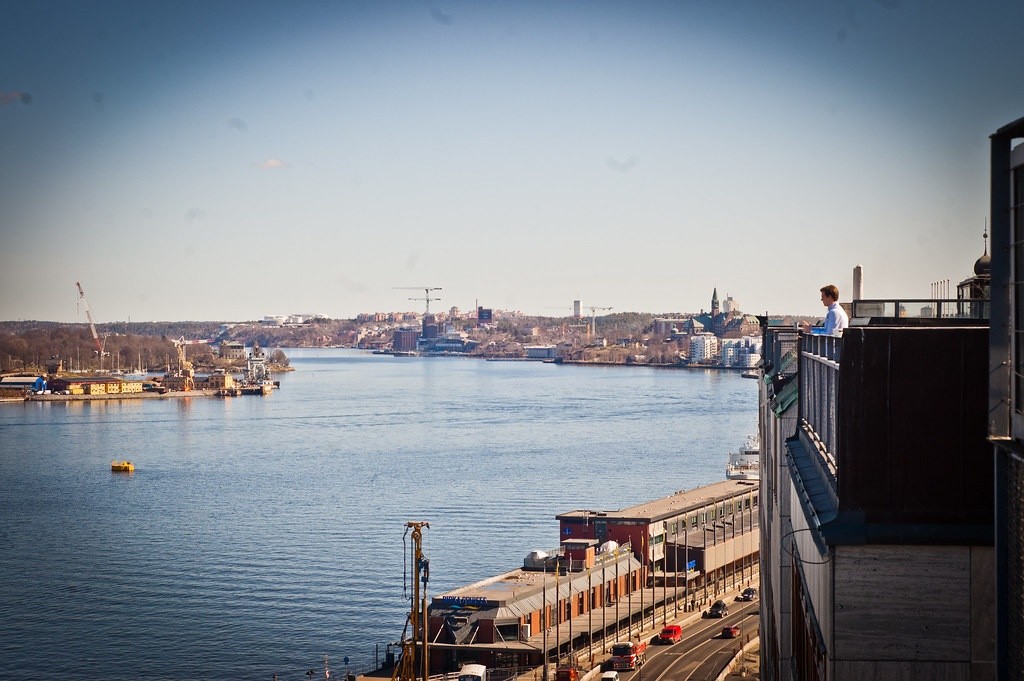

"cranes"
[392,287,442,312]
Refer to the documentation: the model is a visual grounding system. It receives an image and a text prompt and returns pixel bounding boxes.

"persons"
[801,285,849,335]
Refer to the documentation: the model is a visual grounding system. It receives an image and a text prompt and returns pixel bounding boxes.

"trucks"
[608,642,646,671]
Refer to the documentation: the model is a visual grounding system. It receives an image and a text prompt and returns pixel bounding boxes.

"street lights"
[737,595,747,675]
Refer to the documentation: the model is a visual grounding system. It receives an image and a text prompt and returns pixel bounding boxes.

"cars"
[658,625,682,644]
[722,624,741,638]
[741,588,757,600]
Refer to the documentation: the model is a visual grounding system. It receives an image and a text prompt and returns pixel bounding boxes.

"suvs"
[709,600,729,618]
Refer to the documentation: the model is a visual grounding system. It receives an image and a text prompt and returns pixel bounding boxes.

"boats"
[726,417,762,480]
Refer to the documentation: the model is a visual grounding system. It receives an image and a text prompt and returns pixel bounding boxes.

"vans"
[601,671,619,681]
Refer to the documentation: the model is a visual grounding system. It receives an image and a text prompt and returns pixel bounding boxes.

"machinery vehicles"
[76,282,110,357]
